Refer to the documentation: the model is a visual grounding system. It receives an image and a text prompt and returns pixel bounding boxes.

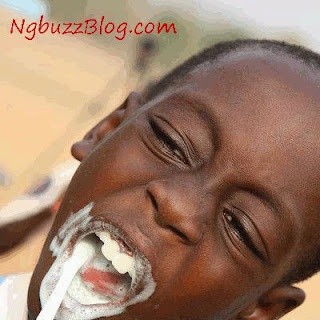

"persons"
[0,38,320,320]
